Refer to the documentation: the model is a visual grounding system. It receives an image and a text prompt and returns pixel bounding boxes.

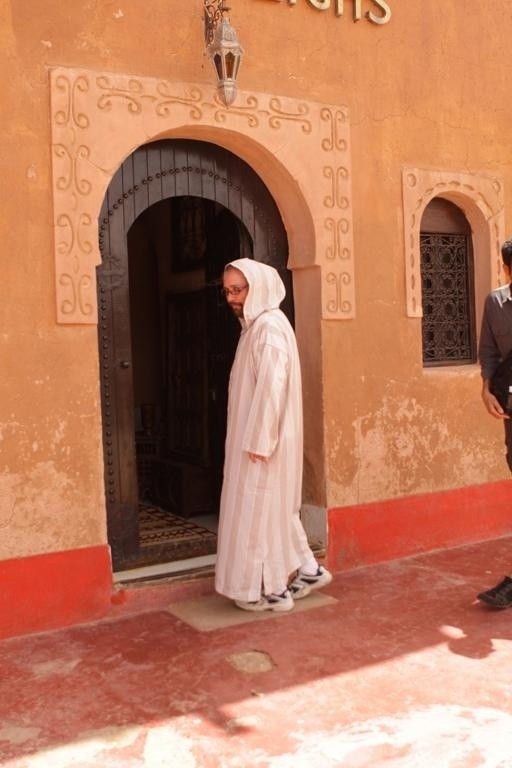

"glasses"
[221,284,248,295]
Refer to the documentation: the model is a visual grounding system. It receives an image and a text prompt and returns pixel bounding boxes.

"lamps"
[201,0,244,110]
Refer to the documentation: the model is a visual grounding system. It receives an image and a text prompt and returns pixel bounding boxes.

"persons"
[474,237,512,613]
[211,257,335,614]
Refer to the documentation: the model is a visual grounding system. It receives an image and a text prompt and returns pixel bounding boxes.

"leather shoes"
[477,576,512,606]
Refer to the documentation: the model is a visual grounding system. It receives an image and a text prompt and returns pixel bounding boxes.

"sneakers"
[234,591,295,612]
[287,563,334,599]
